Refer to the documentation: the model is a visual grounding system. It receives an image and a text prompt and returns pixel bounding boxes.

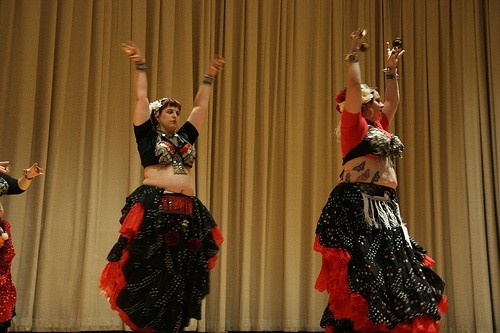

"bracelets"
[22,172,34,180]
[345,52,361,62]
[134,64,148,71]
[202,75,216,84]
[385,73,399,80]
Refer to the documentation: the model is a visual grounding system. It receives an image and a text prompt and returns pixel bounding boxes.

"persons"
[99,40,226,333]
[0,159,45,333]
[313,26,450,333]
